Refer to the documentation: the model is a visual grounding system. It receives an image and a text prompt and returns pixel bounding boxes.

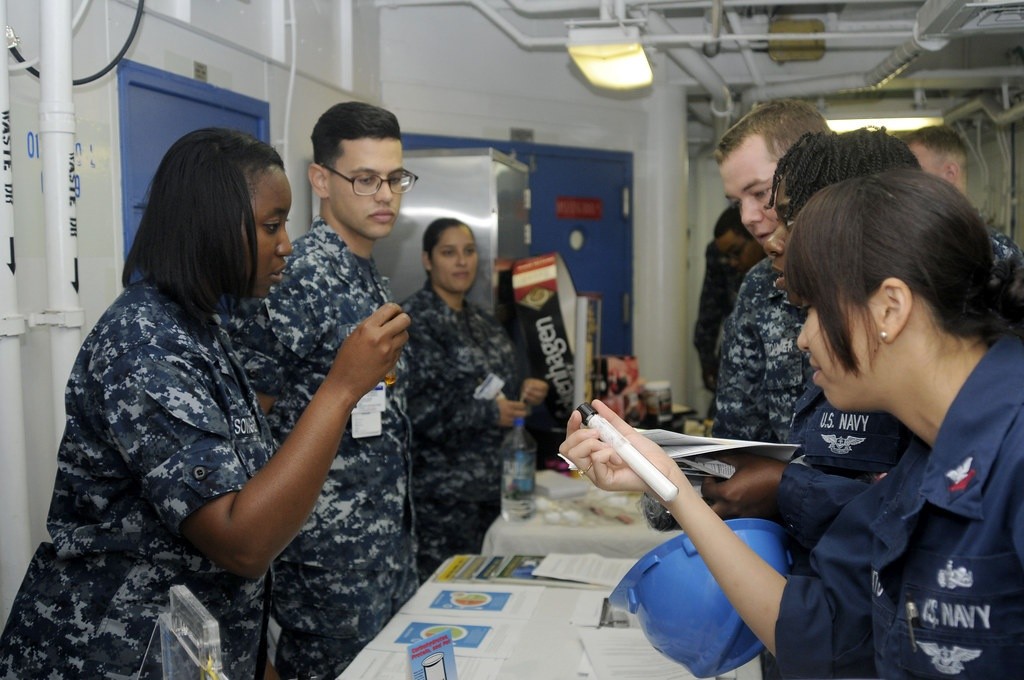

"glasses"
[315,161,419,196]
[718,241,745,265]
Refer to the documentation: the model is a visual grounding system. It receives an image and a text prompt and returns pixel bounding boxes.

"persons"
[898,125,1024,303]
[400,219,549,586]
[700,128,922,577]
[0,127,412,680]
[239,101,420,680]
[710,99,832,444]
[693,200,765,391]
[557,171,1023,680]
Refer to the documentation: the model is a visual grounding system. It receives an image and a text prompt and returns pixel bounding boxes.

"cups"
[643,382,674,422]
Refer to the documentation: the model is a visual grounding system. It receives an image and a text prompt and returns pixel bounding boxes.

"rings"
[577,462,592,477]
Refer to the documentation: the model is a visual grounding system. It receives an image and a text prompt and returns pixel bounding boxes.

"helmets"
[607,517,794,675]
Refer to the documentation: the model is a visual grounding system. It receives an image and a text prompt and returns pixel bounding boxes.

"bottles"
[499,417,538,518]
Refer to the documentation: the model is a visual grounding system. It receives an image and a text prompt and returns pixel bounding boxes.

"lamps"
[565,0,653,90]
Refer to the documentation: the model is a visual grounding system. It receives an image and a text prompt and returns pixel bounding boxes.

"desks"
[486,469,703,559]
[336,554,764,680]
